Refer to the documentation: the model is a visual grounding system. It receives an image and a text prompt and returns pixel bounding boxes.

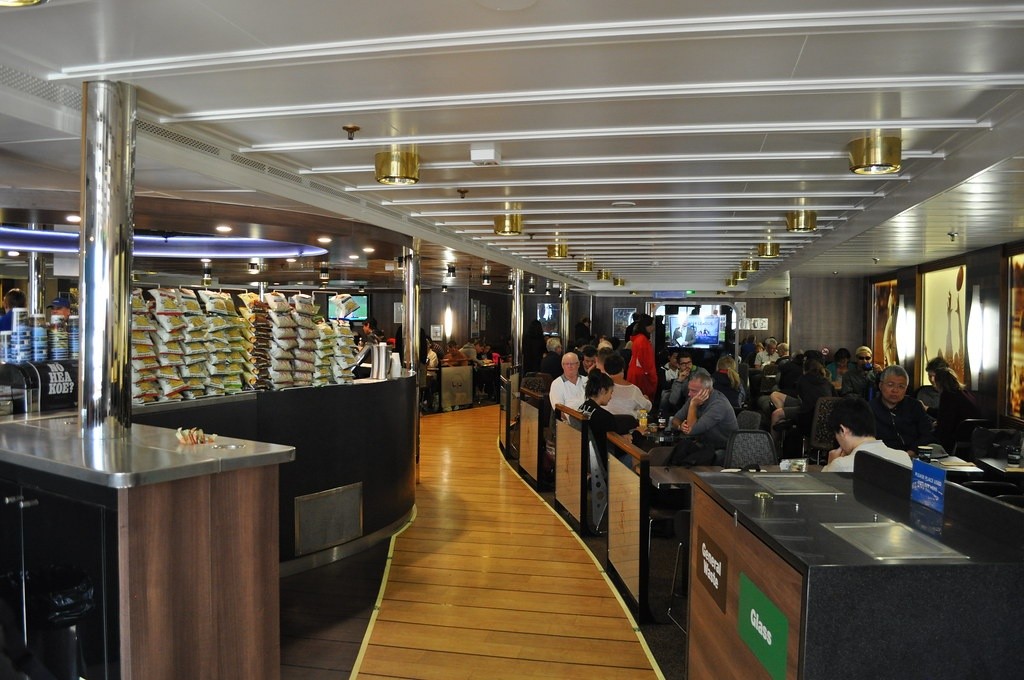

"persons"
[840,345,883,400]
[710,356,747,413]
[866,365,934,458]
[738,334,858,460]
[348,315,501,407]
[543,335,711,415]
[913,355,983,454]
[671,373,739,470]
[580,366,639,475]
[522,320,543,377]
[818,395,914,473]
[574,318,591,344]
[623,313,658,404]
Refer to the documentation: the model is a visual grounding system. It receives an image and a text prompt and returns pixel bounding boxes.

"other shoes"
[490,393,495,402]
[651,526,677,540]
[443,407,453,412]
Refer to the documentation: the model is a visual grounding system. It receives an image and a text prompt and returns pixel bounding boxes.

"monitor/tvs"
[327,293,371,323]
[669,316,721,345]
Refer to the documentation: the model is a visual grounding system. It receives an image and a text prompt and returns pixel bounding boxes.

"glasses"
[679,360,692,365]
[858,356,872,361]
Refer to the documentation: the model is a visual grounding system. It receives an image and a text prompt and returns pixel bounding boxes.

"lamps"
[395,256,405,269]
[373,150,421,186]
[543,288,551,296]
[784,211,818,233]
[199,258,365,294]
[733,271,748,281]
[846,136,903,176]
[441,285,448,293]
[740,261,760,273]
[725,278,738,288]
[446,267,456,279]
[596,271,610,281]
[528,287,535,293]
[757,242,781,259]
[481,277,491,285]
[614,278,625,287]
[578,261,594,274]
[548,243,568,259]
[508,284,514,290]
[494,216,523,238]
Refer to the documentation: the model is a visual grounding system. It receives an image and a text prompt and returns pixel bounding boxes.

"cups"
[0,330,12,364]
[862,364,873,371]
[391,353,401,377]
[1007,448,1021,467]
[47,314,69,361]
[67,315,79,360]
[10,308,32,363]
[919,446,933,464]
[29,314,49,362]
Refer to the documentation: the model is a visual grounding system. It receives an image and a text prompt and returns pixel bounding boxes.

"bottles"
[658,419,666,431]
[639,410,647,430]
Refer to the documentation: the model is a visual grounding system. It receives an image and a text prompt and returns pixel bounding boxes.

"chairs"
[357,327,1024,636]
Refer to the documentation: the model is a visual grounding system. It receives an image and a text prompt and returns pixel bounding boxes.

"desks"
[628,421,693,447]
[911,455,986,483]
[972,455,1024,486]
[648,459,823,602]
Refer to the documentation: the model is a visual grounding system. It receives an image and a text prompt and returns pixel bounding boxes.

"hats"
[46,298,70,309]
